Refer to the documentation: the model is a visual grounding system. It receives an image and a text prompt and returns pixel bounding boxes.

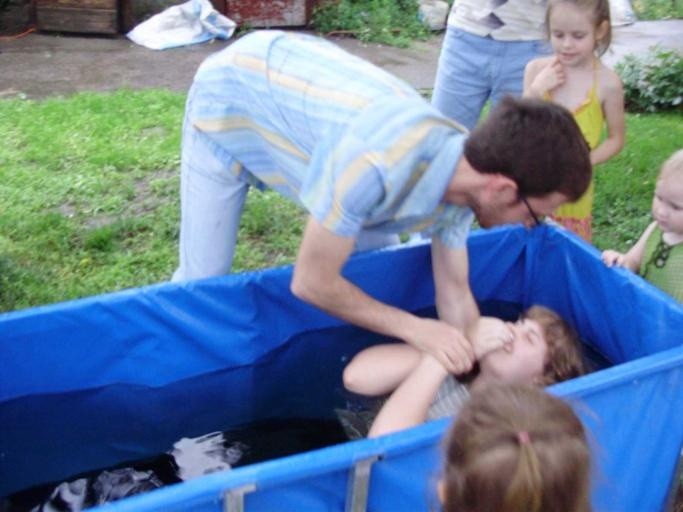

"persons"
[522,0,627,246]
[432,375,593,510]
[0,306,591,511]
[165,26,593,374]
[410,0,555,243]
[601,148,681,308]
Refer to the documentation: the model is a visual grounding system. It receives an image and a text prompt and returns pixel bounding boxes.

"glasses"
[521,197,547,231]
